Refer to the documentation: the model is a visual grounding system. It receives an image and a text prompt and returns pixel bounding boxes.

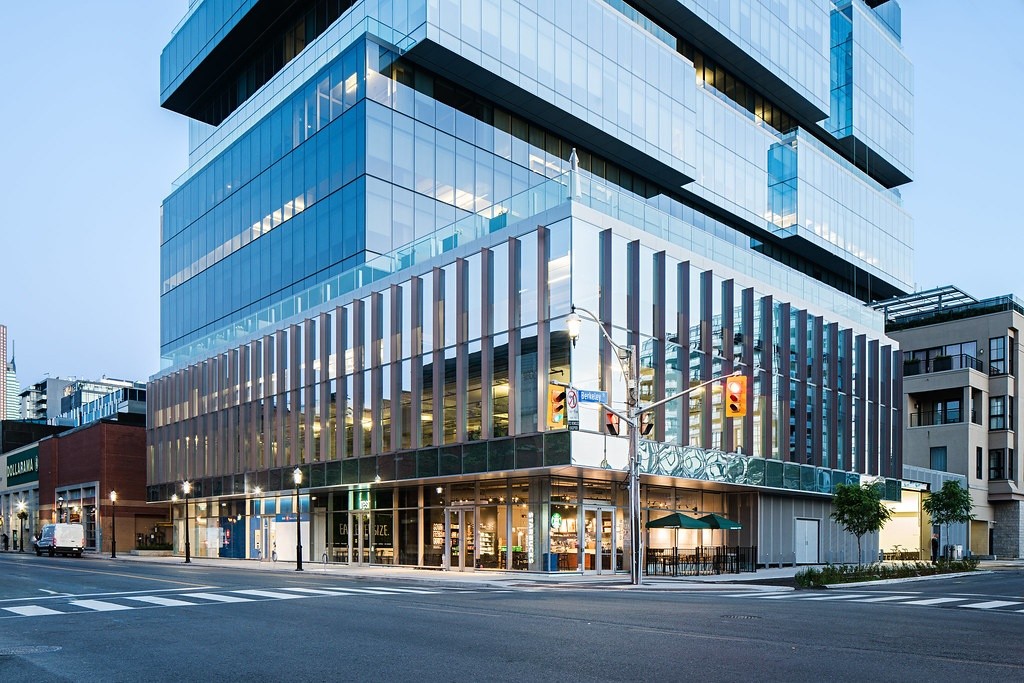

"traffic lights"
[725,376,749,417]
[548,382,566,429]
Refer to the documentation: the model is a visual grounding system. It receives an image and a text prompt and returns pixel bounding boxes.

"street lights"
[183,479,192,563]
[58,497,63,523]
[293,467,304,573]
[18,502,26,554]
[565,306,641,584]
[110,489,118,557]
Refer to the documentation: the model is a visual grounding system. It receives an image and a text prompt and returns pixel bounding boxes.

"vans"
[34,523,83,558]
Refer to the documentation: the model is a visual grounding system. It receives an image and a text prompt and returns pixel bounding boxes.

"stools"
[560,554,570,570]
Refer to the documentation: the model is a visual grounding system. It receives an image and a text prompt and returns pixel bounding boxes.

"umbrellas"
[645,513,711,562]
[696,515,742,561]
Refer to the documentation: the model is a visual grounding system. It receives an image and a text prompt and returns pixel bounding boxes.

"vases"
[389,557,393,564]
[382,558,388,564]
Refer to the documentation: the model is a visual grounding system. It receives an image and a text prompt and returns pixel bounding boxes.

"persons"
[30,529,44,552]
[2,532,8,550]
[932,533,939,565]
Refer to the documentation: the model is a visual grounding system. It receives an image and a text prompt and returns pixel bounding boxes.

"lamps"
[451,498,469,504]
[647,500,689,509]
[694,493,697,515]
